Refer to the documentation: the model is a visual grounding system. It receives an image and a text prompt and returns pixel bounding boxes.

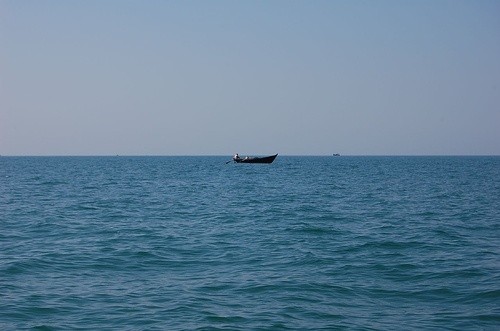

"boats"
[232,153,278,164]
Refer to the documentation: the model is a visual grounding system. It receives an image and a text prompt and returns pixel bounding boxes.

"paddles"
[226,159,234,164]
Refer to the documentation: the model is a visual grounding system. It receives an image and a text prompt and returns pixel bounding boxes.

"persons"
[233,153,240,159]
